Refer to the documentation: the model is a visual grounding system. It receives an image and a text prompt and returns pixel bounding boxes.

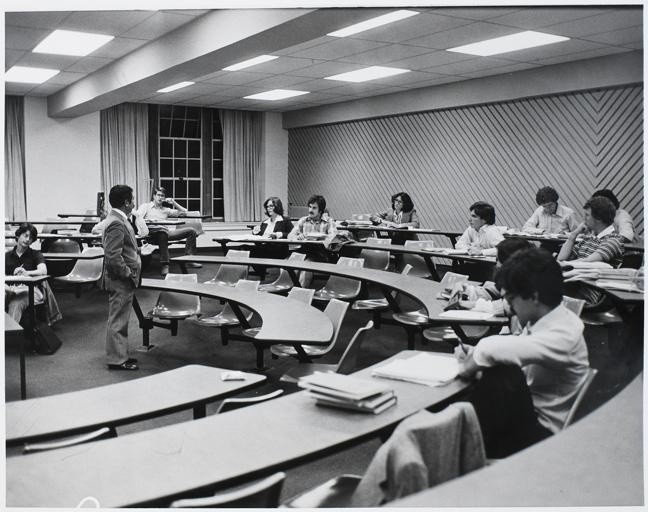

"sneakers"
[108,358,140,371]
[185,260,204,269]
[158,260,170,275]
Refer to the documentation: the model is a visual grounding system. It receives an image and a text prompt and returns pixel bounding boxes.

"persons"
[103,186,144,372]
[521,185,582,257]
[90,210,150,247]
[239,196,294,281]
[454,200,507,280]
[589,189,635,244]
[286,193,337,288]
[369,192,420,245]
[5,221,50,326]
[136,186,203,276]
[452,234,532,334]
[556,197,625,306]
[452,244,590,459]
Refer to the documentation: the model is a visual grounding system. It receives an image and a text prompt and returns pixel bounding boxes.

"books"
[560,257,639,293]
[296,369,400,417]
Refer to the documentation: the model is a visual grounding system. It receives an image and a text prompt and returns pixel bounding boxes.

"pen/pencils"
[162,200,166,201]
[15,263,24,276]
[472,256,485,258]
[458,337,468,355]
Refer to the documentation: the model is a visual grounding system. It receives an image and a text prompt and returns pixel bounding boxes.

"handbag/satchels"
[31,321,62,356]
